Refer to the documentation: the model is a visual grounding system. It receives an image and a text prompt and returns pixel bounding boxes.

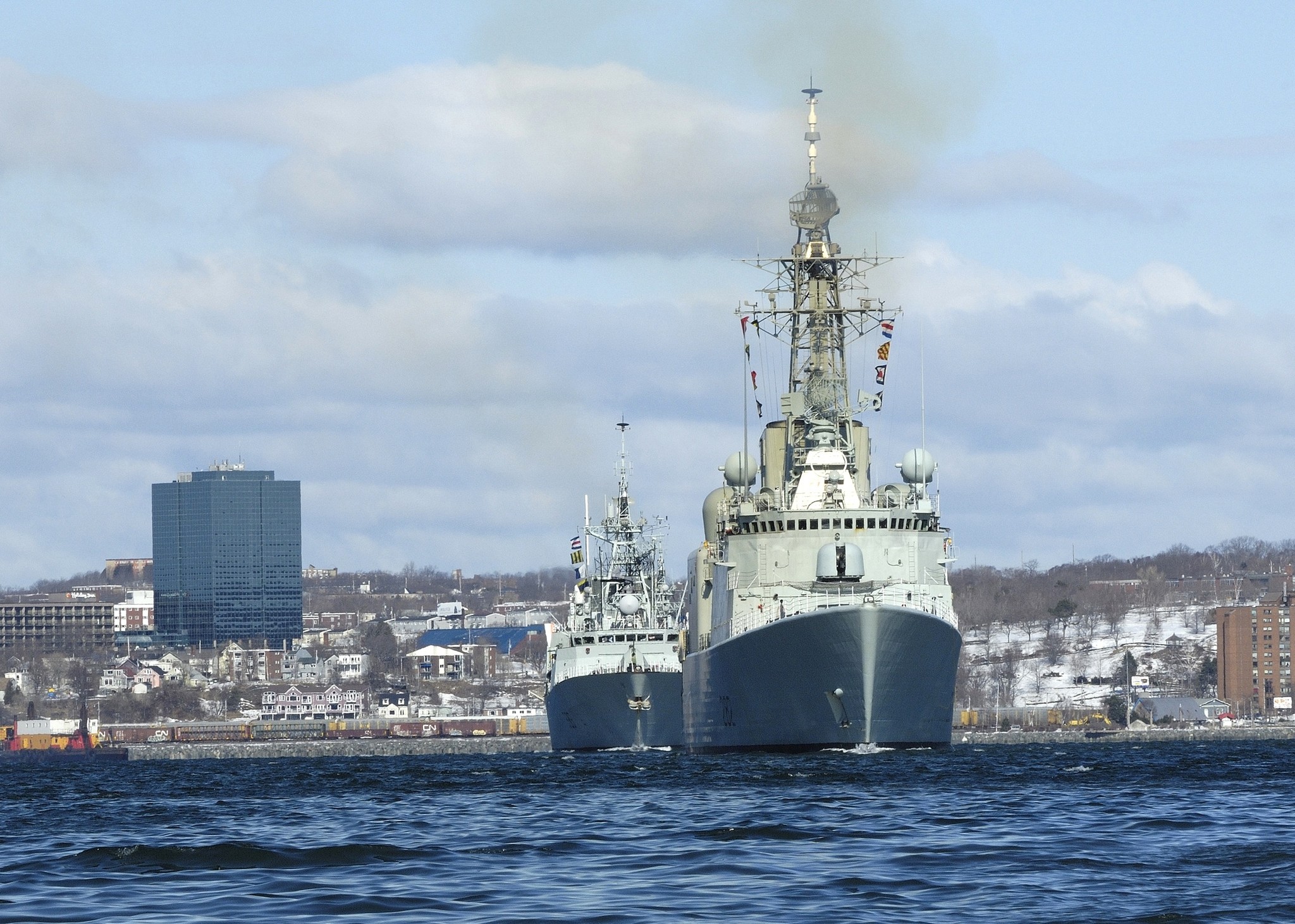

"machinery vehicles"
[1047,709,1114,732]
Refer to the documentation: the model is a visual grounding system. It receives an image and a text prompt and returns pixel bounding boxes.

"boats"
[673,74,965,755]
[540,414,682,753]
[1,700,130,764]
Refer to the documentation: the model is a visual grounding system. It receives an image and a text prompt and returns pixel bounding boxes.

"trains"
[95,714,550,746]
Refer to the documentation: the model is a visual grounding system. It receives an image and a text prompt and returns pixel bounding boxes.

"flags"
[745,344,750,362]
[570,536,582,550]
[875,364,887,385]
[877,341,890,360]
[879,319,895,338]
[576,578,589,595]
[873,391,883,412]
[756,400,763,417]
[741,316,750,338]
[751,320,760,339]
[574,564,587,579]
[751,371,757,391]
[570,550,584,564]
